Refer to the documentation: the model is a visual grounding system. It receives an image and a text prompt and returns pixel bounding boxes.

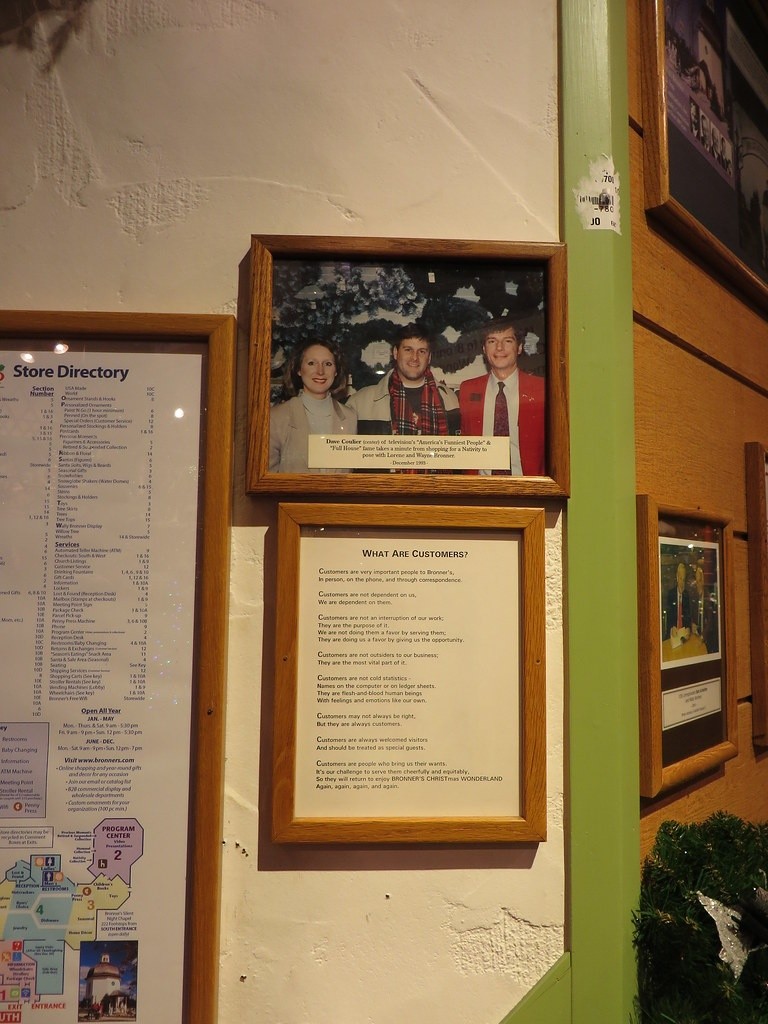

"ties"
[679,595,682,628]
[492,382,512,475]
[697,600,701,635]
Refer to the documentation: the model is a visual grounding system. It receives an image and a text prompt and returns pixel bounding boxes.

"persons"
[666,564,692,641]
[345,323,462,474]
[271,337,359,473]
[689,96,733,176]
[459,317,547,477]
[692,567,717,653]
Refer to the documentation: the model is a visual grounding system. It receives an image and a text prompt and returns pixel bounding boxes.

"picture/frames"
[270,501,547,845]
[635,492,740,798]
[746,441,768,752]
[0,309,234,1024]
[245,233,570,497]
[639,0,768,318]
